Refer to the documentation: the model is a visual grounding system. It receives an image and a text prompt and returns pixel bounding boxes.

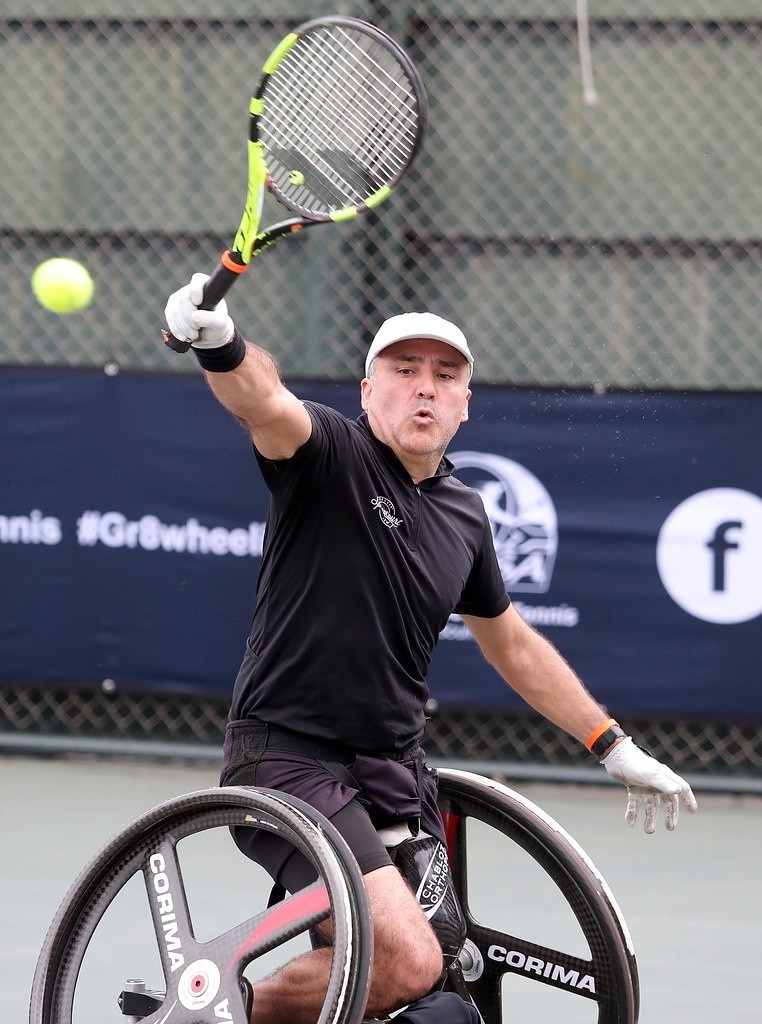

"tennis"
[31,258,96,315]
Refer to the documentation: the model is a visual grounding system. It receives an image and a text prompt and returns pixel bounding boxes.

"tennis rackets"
[162,14,428,353]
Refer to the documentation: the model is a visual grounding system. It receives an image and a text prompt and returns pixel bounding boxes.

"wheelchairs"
[28,767,641,1024]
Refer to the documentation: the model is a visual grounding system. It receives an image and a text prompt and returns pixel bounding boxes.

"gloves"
[165,273,246,373]
[599,735,699,834]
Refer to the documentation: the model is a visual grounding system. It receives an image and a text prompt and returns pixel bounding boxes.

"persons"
[162,271,697,1024]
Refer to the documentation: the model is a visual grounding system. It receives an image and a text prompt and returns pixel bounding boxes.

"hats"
[365,312,473,388]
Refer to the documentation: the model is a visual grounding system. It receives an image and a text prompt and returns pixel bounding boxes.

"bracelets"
[585,719,625,757]
